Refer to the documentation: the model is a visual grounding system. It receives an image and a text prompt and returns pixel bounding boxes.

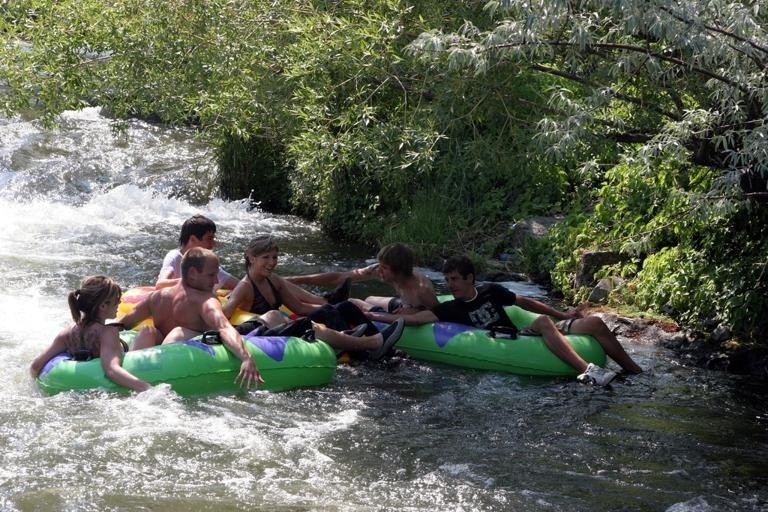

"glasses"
[104,299,121,305]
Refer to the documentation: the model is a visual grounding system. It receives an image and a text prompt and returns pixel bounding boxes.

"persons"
[30,275,208,393]
[280,242,440,316]
[117,247,406,393]
[154,215,353,306]
[219,235,418,371]
[361,255,643,388]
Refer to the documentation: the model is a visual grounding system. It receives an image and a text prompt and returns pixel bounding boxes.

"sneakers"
[577,361,618,387]
[334,321,367,359]
[368,317,406,362]
[322,276,352,306]
